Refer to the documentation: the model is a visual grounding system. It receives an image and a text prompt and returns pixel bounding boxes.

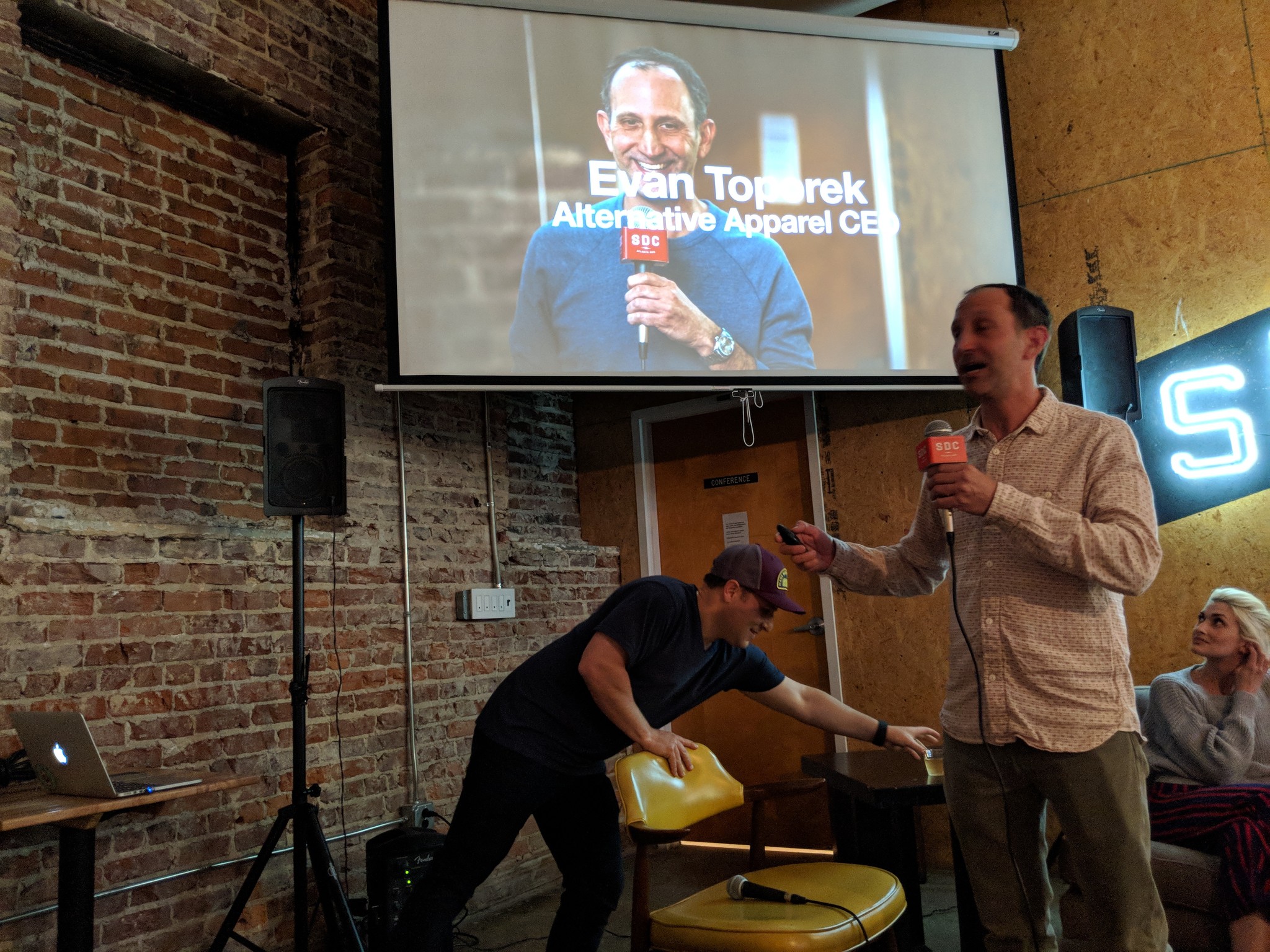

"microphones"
[627,205,657,360]
[726,874,807,905]
[916,419,967,547]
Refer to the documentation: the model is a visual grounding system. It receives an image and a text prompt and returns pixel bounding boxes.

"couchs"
[1055,685,1231,952]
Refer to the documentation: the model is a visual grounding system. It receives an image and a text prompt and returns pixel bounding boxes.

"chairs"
[614,743,908,952]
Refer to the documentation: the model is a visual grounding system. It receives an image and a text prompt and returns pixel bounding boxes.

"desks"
[0,769,260,952]
[799,744,948,952]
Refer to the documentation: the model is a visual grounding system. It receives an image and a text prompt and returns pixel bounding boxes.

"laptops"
[8,711,203,800]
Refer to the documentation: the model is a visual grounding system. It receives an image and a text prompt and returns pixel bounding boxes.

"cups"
[923,749,946,776]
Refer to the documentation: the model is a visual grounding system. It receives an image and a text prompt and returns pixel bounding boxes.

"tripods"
[210,515,366,952]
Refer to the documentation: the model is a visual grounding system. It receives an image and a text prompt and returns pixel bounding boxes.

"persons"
[509,47,814,371]
[1141,588,1270,952]
[775,284,1173,952]
[386,542,940,952]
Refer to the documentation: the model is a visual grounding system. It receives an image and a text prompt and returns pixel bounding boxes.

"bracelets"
[872,720,887,746]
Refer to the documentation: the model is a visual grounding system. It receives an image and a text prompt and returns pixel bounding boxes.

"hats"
[713,543,807,615]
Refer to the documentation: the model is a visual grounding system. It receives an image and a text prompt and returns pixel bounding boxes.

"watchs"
[704,328,735,366]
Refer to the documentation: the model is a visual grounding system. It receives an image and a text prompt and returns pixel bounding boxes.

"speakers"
[263,376,348,516]
[367,827,457,952]
[1057,305,1142,425]
